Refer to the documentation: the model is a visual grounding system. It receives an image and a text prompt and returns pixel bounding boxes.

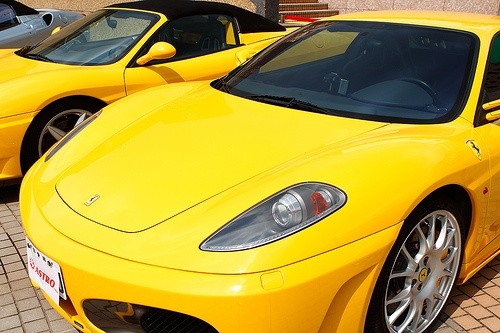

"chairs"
[140,23,174,65]
[323,38,424,98]
[193,29,227,56]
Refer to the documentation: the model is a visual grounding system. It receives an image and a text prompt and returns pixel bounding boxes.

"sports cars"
[2,2,91,50]
[19,11,499,332]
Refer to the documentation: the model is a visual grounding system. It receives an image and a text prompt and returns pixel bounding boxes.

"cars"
[0,1,309,182]
